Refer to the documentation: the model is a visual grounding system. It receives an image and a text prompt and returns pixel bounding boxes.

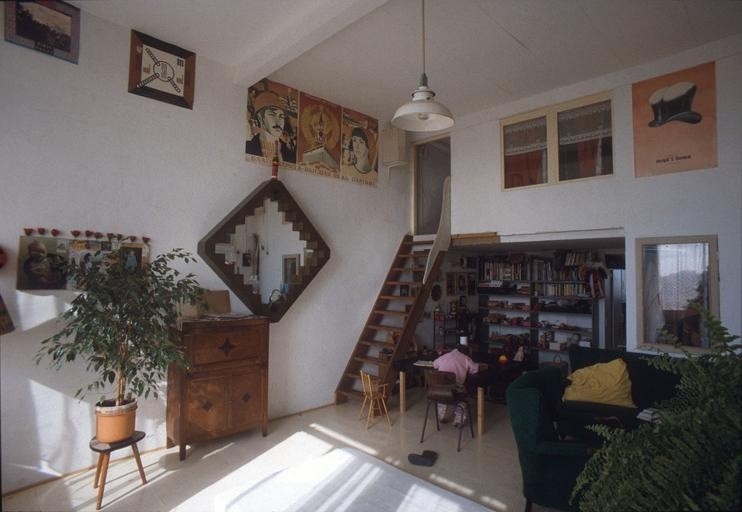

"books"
[477,246,596,298]
[637,407,664,426]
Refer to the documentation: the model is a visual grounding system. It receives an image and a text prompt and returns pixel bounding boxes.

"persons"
[431,344,480,428]
[340,126,379,186]
[245,89,296,164]
[22,237,141,290]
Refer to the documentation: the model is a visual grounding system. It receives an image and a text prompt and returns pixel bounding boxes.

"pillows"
[560,359,640,408]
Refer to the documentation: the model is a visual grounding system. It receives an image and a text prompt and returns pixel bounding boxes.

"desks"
[393,350,500,434]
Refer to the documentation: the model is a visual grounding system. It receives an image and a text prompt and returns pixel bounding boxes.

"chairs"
[420,370,476,452]
[506,363,646,512]
[355,371,393,430]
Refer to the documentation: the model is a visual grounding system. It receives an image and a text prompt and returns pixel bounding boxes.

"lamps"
[389,2,457,133]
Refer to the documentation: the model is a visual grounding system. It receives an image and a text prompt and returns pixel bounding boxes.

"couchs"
[568,343,700,417]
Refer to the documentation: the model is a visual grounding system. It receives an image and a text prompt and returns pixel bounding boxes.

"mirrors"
[634,234,721,357]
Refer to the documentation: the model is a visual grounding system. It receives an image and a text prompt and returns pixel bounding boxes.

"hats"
[252,91,287,118]
[647,81,703,129]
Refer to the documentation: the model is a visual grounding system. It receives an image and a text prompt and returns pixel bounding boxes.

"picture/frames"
[128,28,197,110]
[0,0,81,64]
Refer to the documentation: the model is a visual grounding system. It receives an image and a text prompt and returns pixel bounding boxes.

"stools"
[88,432,148,510]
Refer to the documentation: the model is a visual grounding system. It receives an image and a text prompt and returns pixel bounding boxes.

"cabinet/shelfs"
[478,254,598,403]
[166,314,270,461]
[433,311,458,346]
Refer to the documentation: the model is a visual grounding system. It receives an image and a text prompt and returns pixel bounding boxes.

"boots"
[408,450,438,467]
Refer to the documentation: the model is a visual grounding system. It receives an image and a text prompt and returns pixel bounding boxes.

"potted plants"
[32,235,215,444]
